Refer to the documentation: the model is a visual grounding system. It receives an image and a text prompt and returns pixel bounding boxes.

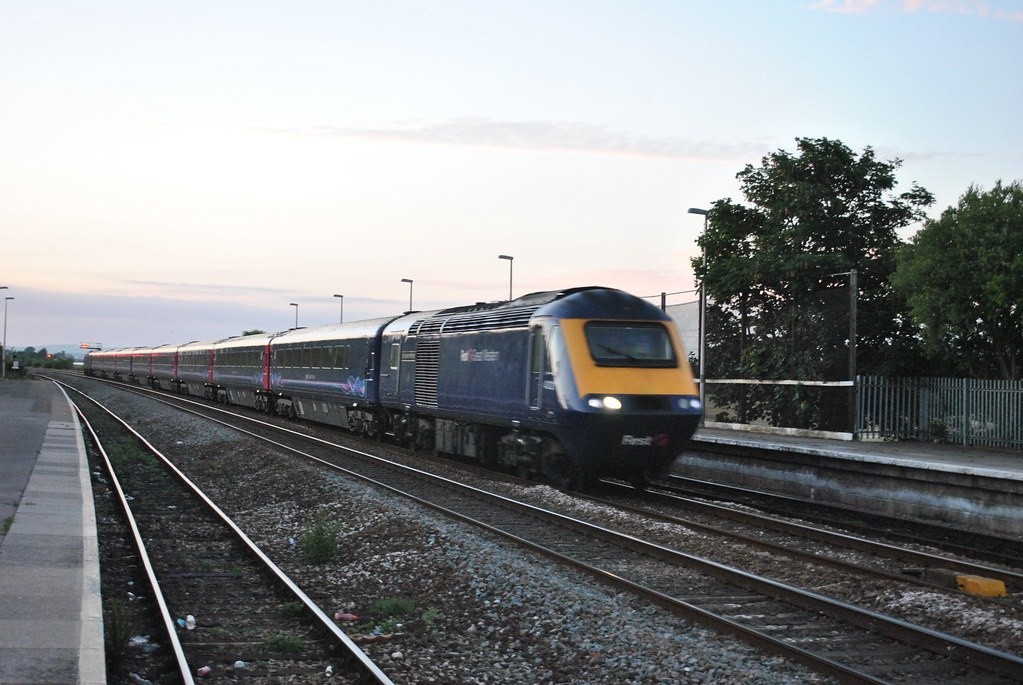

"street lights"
[499,255,513,301]
[334,294,343,324]
[2,297,14,379]
[401,279,413,312]
[686,208,709,288]
[290,303,298,328]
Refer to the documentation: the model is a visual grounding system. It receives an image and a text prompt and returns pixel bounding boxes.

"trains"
[83,285,704,492]
